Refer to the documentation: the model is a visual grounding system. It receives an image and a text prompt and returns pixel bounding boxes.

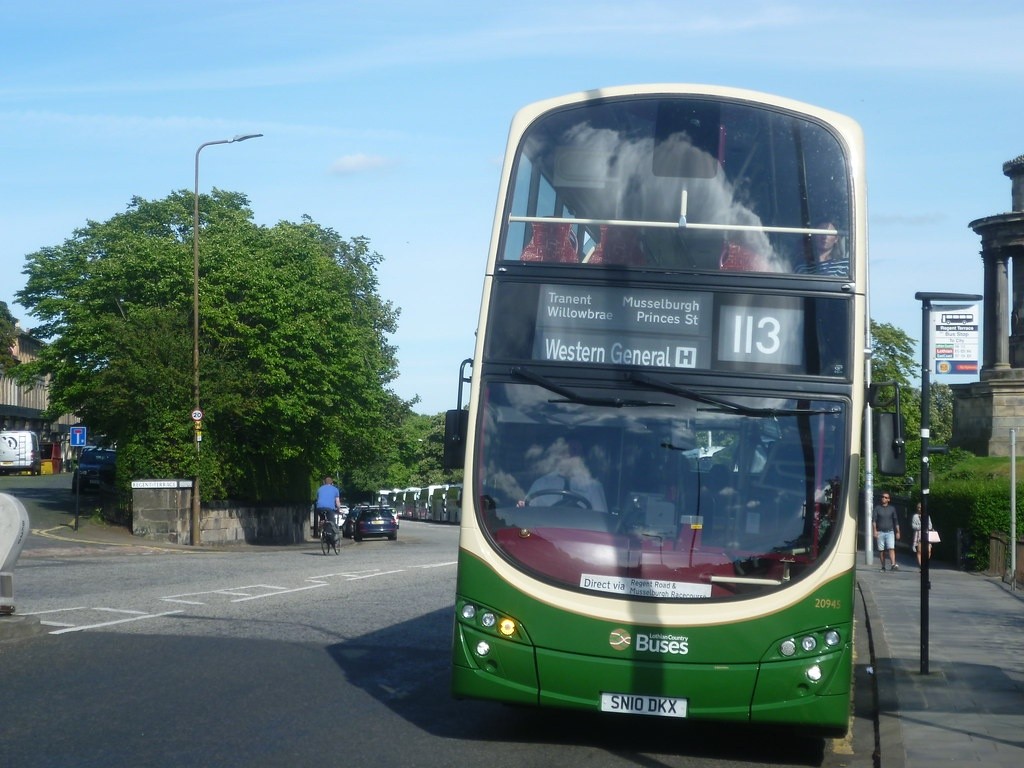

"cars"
[334,503,400,536]
[350,508,398,542]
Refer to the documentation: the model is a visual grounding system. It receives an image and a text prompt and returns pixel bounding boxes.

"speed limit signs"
[191,410,203,422]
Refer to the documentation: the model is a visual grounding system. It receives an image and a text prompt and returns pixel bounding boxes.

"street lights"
[190,132,265,547]
[912,290,987,676]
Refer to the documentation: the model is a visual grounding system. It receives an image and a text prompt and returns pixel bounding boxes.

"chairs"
[520,215,772,274]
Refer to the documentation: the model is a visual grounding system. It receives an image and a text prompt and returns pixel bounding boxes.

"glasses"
[882,496,890,499]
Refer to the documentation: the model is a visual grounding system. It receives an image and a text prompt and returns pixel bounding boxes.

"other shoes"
[891,565,899,570]
[880,567,885,573]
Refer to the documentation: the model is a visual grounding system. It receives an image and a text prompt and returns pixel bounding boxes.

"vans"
[71,444,118,495]
[0,430,43,474]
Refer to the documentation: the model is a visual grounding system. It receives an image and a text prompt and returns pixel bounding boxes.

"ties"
[558,473,571,500]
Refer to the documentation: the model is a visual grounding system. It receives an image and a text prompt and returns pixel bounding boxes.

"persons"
[315,477,343,550]
[911,502,933,573]
[872,492,901,572]
[794,219,853,280]
[514,432,609,515]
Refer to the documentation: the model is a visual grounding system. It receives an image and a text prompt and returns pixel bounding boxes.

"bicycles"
[318,506,347,556]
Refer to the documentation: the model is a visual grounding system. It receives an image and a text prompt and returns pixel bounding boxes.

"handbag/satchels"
[928,529,941,543]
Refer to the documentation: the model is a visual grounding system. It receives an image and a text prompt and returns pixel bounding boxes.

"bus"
[439,82,909,740]
